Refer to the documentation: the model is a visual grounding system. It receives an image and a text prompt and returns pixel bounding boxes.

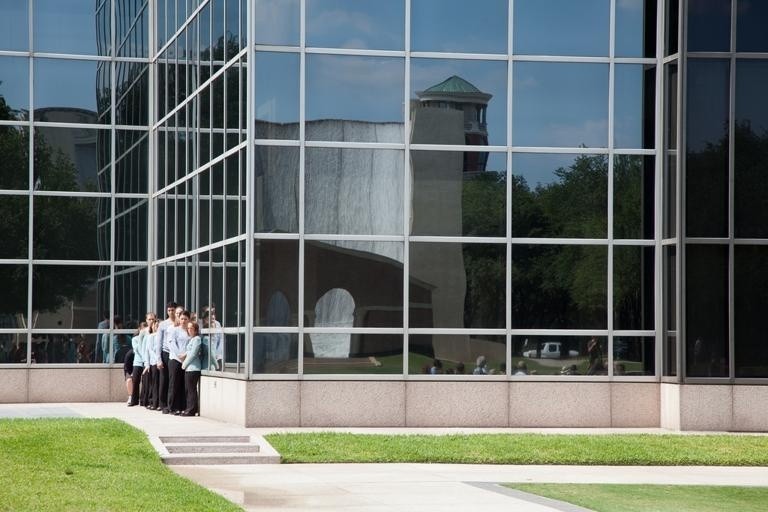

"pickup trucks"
[523,340,581,359]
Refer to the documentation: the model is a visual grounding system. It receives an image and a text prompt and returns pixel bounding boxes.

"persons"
[186,302,225,371]
[425,334,627,376]
[0,309,140,364]
[122,301,203,417]
